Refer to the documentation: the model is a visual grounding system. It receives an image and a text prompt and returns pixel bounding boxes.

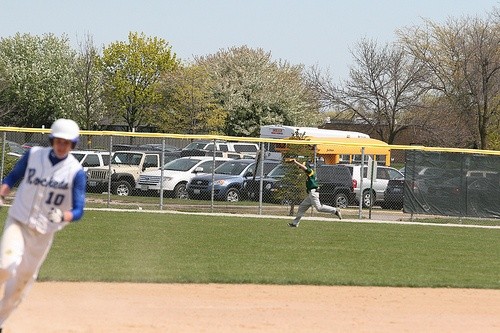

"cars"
[340,164,500,211]
[1,139,281,204]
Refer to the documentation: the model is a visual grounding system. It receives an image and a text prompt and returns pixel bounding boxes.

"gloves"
[48,208,64,224]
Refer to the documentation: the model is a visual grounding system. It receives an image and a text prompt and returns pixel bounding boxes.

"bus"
[260,124,392,166]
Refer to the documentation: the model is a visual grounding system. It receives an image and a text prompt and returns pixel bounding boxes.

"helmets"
[49,119,80,144]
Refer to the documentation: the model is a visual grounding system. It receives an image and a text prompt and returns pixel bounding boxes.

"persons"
[285,158,342,227]
[0,119,88,333]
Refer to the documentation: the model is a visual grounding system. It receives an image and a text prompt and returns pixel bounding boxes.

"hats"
[299,162,308,168]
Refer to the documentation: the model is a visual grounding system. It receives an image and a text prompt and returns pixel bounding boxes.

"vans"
[241,163,357,210]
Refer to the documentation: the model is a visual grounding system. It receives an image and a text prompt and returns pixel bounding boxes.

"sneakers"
[288,223,298,227]
[335,209,342,219]
